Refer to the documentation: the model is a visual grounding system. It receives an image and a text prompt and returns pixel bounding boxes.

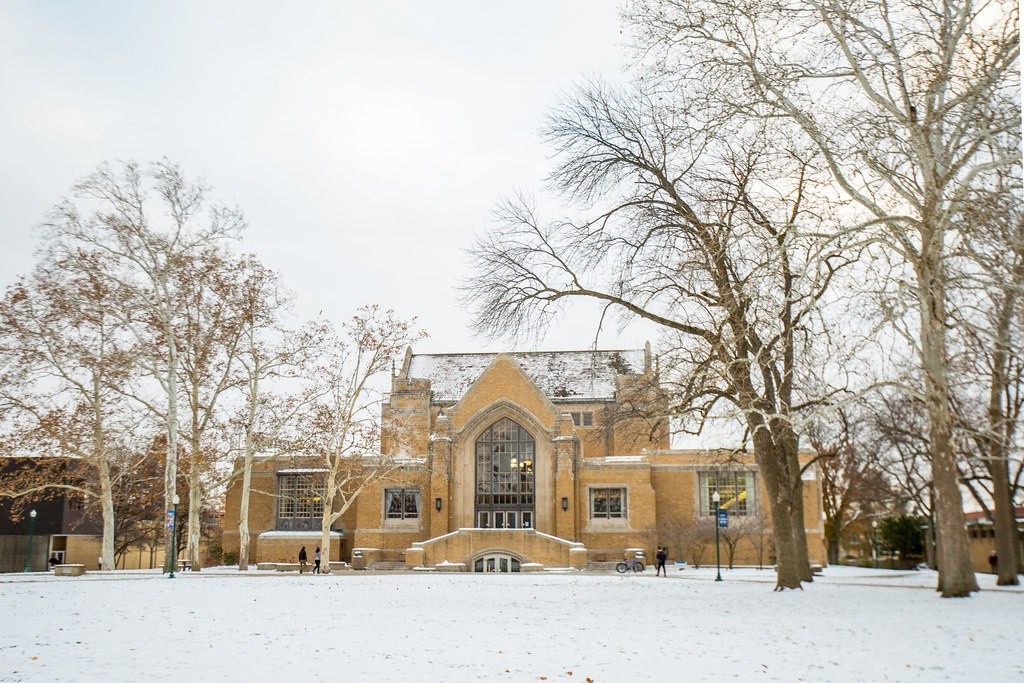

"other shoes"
[313,570,314,573]
[318,571,319,573]
[664,575,666,577]
[300,570,302,573]
[656,575,659,576]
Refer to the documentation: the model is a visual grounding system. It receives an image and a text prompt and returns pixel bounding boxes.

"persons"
[989,550,998,574]
[98,556,102,570]
[298,546,307,574]
[49,555,62,566]
[656,546,667,577]
[312,546,322,574]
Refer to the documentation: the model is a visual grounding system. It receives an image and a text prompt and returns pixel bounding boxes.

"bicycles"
[615,553,644,572]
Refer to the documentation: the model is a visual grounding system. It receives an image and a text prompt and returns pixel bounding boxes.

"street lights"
[168,494,180,578]
[713,489,724,580]
[24,508,37,571]
[871,521,879,568]
